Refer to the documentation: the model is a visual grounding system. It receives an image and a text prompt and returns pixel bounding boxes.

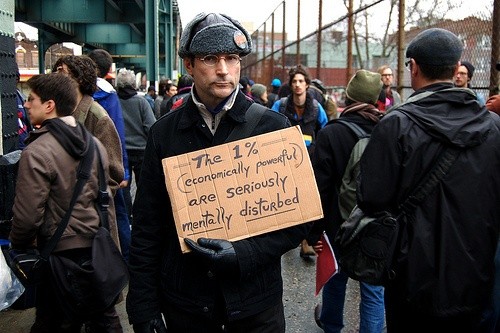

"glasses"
[194,54,242,67]
[405,58,418,71]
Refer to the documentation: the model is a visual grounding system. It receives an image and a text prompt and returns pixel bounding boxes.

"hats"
[148,86,156,92]
[178,12,251,56]
[459,61,474,80]
[271,79,281,88]
[406,28,463,65]
[346,69,383,107]
[251,84,266,98]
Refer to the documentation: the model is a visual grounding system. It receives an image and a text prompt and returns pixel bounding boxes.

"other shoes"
[315,304,327,333]
[300,240,316,256]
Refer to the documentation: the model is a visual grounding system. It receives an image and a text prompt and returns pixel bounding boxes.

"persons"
[2,73,130,333]
[308,69,395,333]
[65,45,500,264]
[45,55,127,308]
[127,9,319,333]
[355,26,500,333]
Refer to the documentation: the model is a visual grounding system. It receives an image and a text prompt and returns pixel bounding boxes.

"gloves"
[184,238,240,278]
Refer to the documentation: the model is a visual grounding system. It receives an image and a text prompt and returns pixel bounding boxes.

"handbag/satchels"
[332,205,399,283]
[4,248,47,310]
[40,227,129,322]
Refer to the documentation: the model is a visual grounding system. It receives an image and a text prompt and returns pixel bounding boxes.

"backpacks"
[337,119,372,220]
